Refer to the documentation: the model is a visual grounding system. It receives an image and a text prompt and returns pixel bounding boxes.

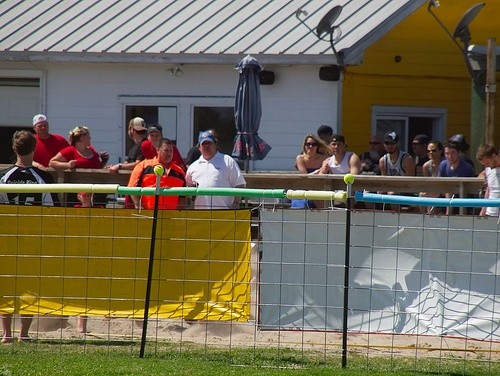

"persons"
[32,113,70,171]
[0,129,61,207]
[294,125,500,217]
[48,124,110,208]
[107,117,247,210]
[74,192,105,208]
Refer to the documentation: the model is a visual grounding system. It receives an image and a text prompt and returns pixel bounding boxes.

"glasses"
[426,150,438,153]
[305,142,317,146]
[384,141,397,145]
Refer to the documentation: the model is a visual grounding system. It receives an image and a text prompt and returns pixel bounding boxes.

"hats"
[32,114,47,127]
[149,127,161,136]
[414,134,430,144]
[449,134,469,150]
[384,132,400,142]
[198,130,217,144]
[330,134,348,148]
[128,117,148,132]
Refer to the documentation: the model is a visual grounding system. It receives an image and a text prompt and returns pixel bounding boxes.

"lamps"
[167,63,184,77]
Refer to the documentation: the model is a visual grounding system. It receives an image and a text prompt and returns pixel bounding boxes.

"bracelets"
[118,163,122,169]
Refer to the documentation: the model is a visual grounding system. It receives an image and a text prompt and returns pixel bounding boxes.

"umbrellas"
[232,54,271,173]
